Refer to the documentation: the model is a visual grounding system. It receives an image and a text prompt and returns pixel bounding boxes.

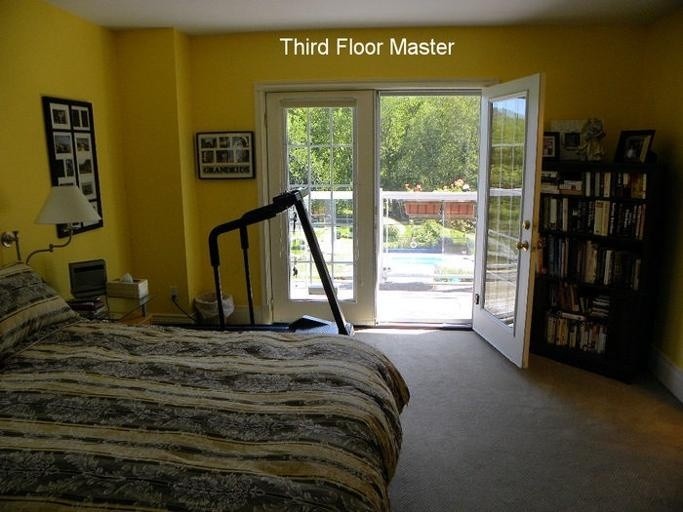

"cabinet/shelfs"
[529,118,658,388]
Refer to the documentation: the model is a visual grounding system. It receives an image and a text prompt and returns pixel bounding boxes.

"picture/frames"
[40,92,104,240]
[195,129,256,181]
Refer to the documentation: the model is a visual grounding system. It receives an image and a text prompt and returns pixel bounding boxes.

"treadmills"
[149,187,354,337]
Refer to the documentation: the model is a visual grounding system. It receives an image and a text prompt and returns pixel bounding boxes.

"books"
[64,299,114,323]
[533,164,647,359]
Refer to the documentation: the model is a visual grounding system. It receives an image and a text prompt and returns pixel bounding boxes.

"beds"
[0,261,411,512]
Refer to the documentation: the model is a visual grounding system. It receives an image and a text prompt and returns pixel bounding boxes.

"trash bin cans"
[194,291,233,325]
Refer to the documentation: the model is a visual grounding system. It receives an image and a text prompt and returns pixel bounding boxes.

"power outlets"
[169,285,176,297]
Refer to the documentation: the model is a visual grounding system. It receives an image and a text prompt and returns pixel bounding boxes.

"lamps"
[1,184,103,265]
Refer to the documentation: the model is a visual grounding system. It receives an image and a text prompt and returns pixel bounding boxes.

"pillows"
[0,263,90,366]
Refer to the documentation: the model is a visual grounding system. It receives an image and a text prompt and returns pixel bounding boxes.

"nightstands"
[114,311,152,326]
